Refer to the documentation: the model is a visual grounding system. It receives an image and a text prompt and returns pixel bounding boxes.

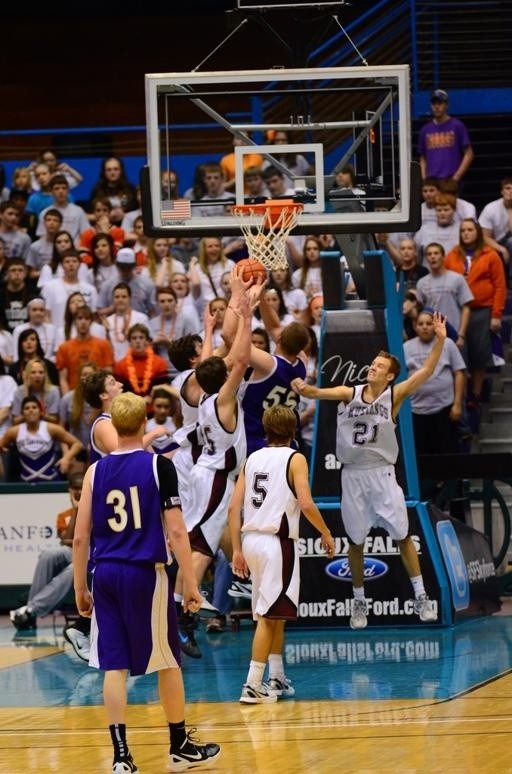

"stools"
[54,581,82,639]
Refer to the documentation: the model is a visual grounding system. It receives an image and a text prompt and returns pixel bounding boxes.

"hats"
[429,89,448,104]
[115,248,137,266]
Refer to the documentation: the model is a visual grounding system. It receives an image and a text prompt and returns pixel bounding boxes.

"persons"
[0,93,512,704]
[72,392,220,773]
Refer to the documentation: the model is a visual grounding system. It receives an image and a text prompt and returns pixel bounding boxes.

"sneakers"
[350,599,370,630]
[206,615,226,633]
[239,682,279,705]
[267,676,296,698]
[227,580,252,600]
[412,594,438,623]
[180,591,220,619]
[466,393,481,408]
[175,623,203,659]
[111,756,138,774]
[62,624,91,663]
[9,605,35,631]
[167,736,222,774]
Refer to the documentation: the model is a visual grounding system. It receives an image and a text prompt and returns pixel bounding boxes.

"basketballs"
[234,258,267,287]
